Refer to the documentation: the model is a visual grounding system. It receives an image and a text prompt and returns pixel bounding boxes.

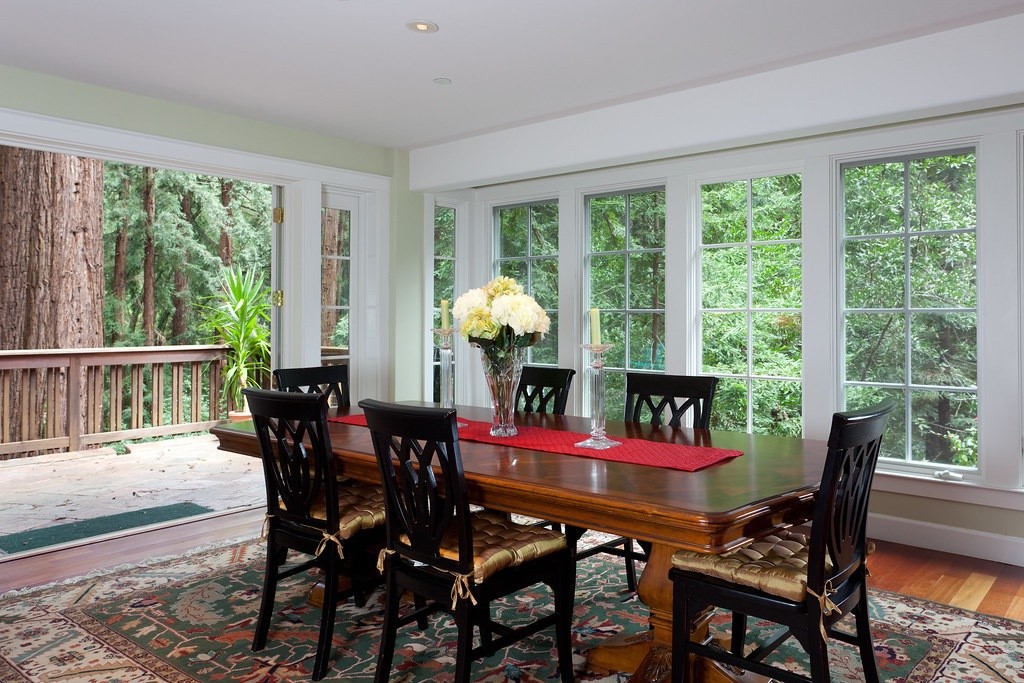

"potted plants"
[191,262,272,422]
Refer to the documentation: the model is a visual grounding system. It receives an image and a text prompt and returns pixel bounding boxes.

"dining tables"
[209,404,830,683]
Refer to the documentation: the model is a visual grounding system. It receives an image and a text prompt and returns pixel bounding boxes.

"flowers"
[451,275,551,349]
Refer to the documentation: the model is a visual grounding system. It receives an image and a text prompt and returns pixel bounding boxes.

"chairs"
[513,365,576,533]
[240,386,428,682]
[356,399,577,683]
[668,395,896,683]
[274,363,352,408]
[564,372,719,592]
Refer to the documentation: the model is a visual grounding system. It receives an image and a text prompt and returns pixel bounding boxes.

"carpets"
[0,500,1024,683]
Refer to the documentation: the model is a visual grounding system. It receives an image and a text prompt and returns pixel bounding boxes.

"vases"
[481,349,524,437]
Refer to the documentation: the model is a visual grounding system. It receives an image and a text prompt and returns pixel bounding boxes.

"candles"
[441,299,450,330]
[590,308,601,345]
